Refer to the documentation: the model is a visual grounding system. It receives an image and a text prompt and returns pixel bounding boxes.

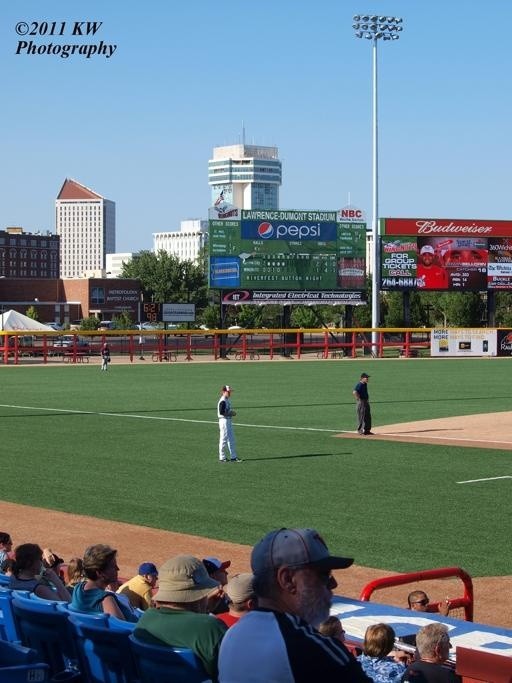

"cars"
[45,318,246,330]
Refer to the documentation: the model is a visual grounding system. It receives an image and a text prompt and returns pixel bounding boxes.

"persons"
[216,384,244,462]
[414,244,448,288]
[447,248,488,264]
[101,342,110,370]
[353,372,373,434]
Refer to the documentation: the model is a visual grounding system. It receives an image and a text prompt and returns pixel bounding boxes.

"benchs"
[315,347,344,359]
[233,348,261,361]
[151,350,177,362]
[62,352,90,363]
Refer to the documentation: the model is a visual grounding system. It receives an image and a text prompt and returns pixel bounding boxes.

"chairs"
[0,573,206,682]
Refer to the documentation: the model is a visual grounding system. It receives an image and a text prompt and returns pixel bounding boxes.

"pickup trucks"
[53,333,90,353]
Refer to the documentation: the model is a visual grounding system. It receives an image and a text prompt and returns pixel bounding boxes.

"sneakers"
[219,457,245,463]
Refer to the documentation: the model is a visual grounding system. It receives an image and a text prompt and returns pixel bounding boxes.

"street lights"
[350,13,404,356]
[102,271,112,318]
[0,275,5,278]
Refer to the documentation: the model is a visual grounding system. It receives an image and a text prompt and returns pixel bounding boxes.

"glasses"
[412,599,429,606]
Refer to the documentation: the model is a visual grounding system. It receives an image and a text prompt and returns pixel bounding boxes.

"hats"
[252,527,355,575]
[138,562,158,576]
[42,553,65,569]
[361,372,369,378]
[221,385,233,393]
[151,552,231,599]
